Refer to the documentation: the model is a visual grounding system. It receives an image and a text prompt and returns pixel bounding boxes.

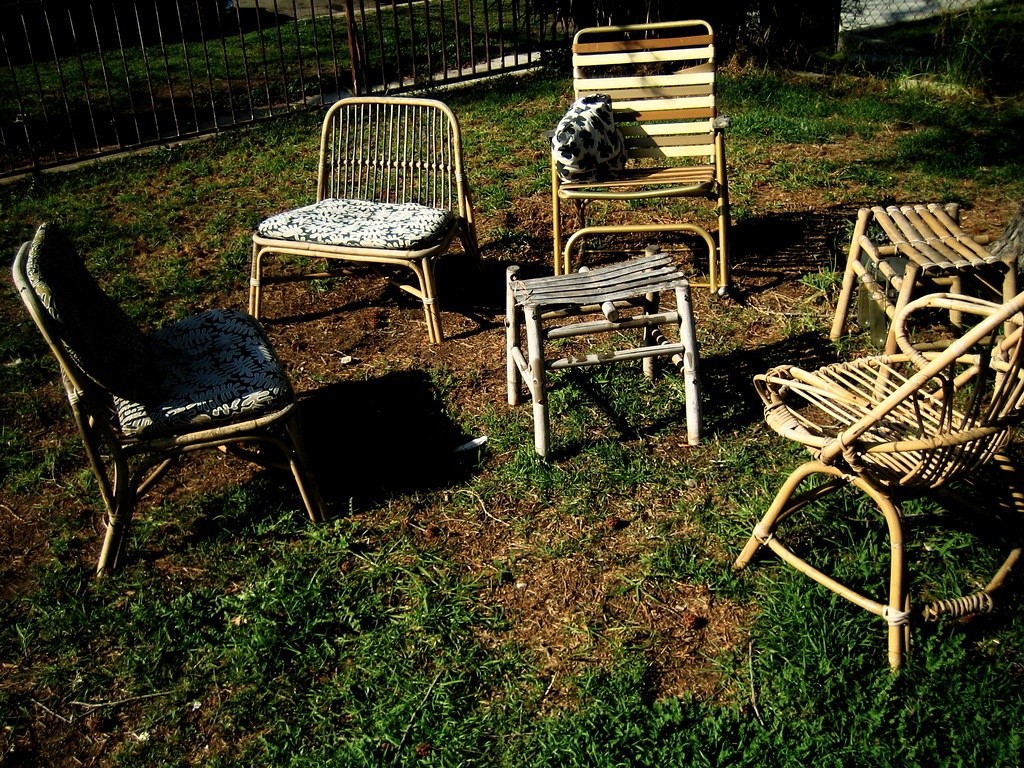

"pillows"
[553,92,628,187]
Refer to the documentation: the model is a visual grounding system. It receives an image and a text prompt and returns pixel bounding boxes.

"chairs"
[248,96,479,344]
[12,222,328,578]
[553,19,731,293]
[732,291,1024,670]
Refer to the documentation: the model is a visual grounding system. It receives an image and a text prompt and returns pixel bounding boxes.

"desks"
[829,202,1018,398]
[503,246,702,455]
